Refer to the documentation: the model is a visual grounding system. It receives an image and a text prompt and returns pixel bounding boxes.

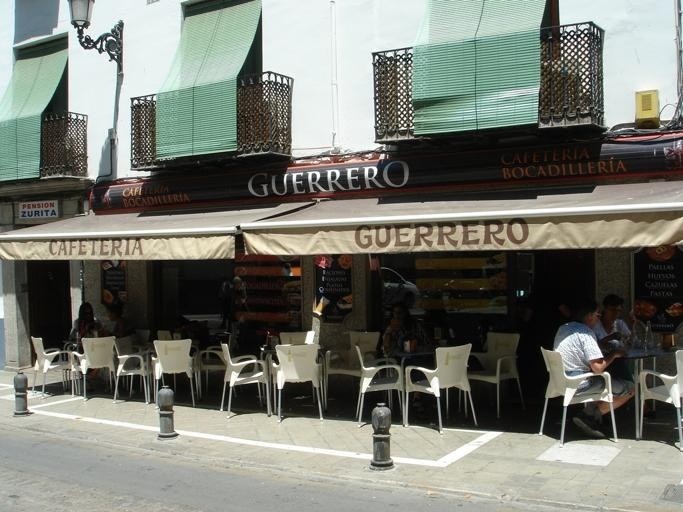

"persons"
[68,300,104,344]
[595,293,655,417]
[86,304,126,391]
[380,301,435,419]
[552,298,633,439]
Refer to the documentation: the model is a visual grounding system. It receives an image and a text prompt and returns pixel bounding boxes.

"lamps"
[66,1,124,78]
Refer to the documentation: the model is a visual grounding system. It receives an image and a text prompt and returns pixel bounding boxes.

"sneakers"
[582,416,606,438]
[572,411,591,428]
